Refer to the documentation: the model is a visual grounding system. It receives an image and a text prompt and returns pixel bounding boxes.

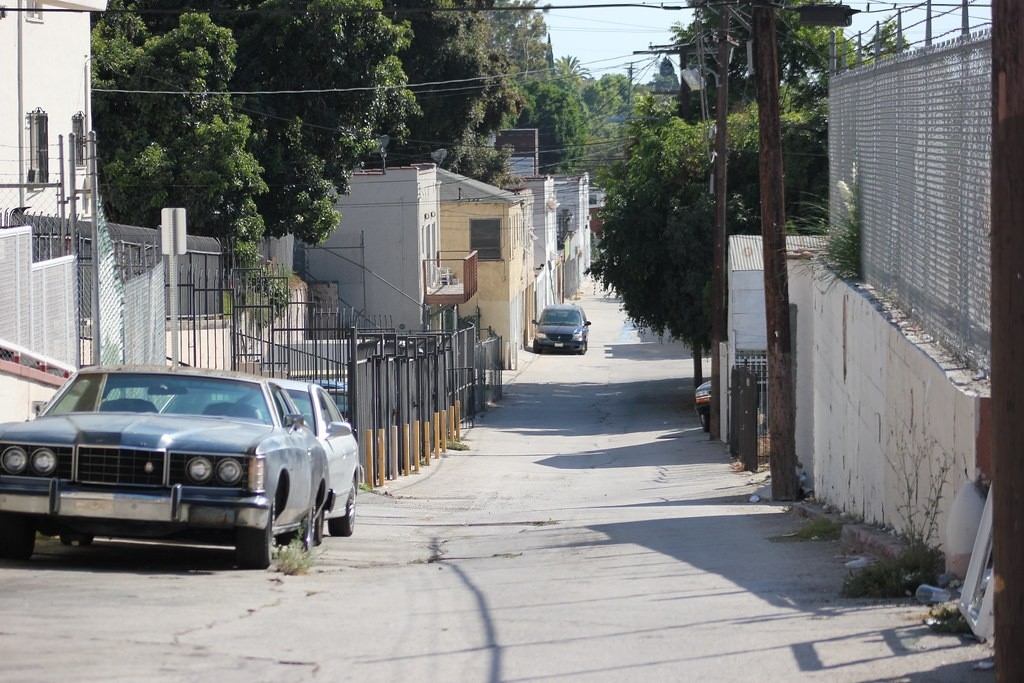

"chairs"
[204,402,261,418]
[101,398,157,412]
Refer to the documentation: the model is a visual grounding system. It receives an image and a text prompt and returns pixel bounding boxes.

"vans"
[531,304,591,355]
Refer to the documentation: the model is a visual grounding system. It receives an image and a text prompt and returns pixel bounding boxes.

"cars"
[0,364,331,571]
[694,380,713,433]
[156,377,360,546]
[298,378,348,422]
